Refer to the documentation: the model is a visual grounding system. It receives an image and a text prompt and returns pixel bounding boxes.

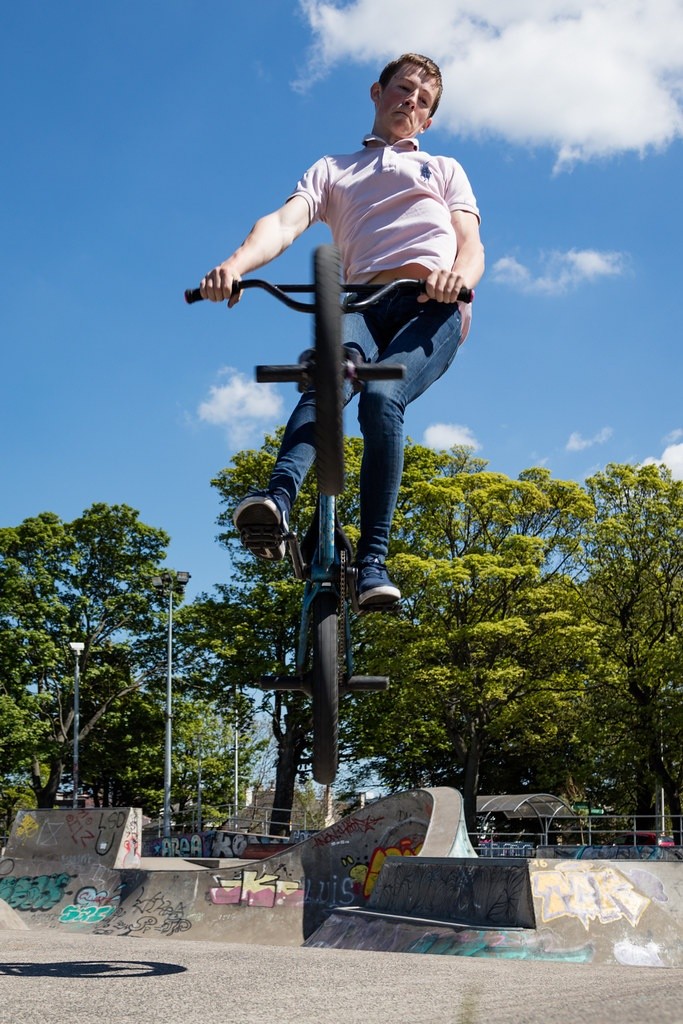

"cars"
[622,831,675,847]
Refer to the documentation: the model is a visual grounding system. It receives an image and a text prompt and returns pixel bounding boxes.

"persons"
[200,52,485,606]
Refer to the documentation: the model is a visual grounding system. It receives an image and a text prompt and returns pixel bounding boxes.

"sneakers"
[356,555,401,605]
[232,483,287,561]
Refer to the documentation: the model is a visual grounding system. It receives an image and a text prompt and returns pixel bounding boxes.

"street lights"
[68,641,87,808]
[151,570,193,847]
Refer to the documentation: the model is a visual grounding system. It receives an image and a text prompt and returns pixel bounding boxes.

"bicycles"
[183,240,475,783]
